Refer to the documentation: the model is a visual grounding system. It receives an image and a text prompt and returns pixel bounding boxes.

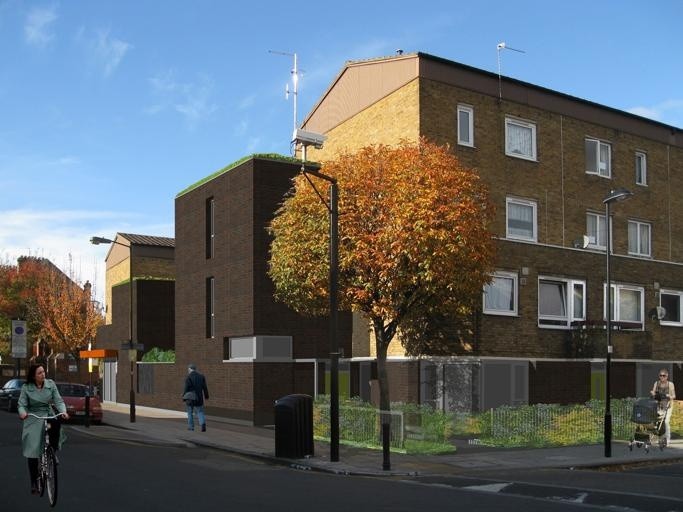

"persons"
[181,364,209,432]
[649,368,676,447]
[17,363,71,495]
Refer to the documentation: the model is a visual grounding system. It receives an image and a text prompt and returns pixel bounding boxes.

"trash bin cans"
[274,393,313,458]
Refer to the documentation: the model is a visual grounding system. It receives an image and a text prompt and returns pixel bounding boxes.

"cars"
[0,379,103,420]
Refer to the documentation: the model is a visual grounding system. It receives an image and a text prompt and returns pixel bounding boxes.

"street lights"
[602,190,633,457]
[89,236,135,422]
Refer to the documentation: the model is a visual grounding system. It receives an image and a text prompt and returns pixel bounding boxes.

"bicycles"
[24,413,66,506]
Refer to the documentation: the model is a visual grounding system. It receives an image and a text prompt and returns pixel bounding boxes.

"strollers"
[629,395,671,453]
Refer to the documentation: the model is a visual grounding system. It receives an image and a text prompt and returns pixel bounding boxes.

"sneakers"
[189,423,206,432]
[31,485,40,495]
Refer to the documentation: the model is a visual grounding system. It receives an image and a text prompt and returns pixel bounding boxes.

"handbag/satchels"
[183,392,198,405]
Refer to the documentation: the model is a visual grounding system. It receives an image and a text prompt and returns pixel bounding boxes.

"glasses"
[660,375,666,377]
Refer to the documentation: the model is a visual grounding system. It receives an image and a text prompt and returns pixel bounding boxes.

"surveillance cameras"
[292,129,325,149]
